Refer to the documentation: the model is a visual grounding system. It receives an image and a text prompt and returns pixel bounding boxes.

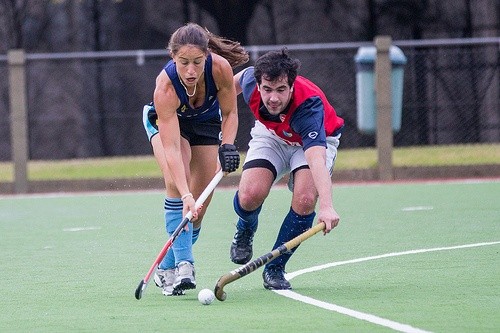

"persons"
[143,21,249,297]
[231,47,345,290]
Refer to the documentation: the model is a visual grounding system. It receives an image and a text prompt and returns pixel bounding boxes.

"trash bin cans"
[353,46,408,135]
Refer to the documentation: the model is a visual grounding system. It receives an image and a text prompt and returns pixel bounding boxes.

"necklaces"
[178,74,197,97]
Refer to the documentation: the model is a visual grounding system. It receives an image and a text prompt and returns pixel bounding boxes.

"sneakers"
[172,260,197,290]
[262,264,294,291]
[229,221,258,265]
[153,268,186,296]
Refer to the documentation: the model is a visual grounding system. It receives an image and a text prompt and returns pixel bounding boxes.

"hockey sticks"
[214,221,326,302]
[134,168,224,301]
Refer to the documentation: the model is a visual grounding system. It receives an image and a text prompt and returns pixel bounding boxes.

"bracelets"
[180,193,193,201]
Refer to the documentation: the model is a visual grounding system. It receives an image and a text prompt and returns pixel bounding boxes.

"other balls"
[198,289,215,305]
